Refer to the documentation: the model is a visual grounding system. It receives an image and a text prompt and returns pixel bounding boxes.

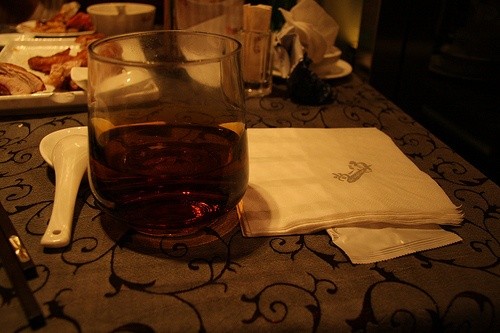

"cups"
[85,29,250,254]
[163,0,244,43]
[241,30,273,99]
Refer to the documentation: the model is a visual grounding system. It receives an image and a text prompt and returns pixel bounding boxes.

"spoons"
[40,134,88,248]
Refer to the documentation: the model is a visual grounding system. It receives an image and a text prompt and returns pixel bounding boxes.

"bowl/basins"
[315,47,343,74]
[87,3,156,36]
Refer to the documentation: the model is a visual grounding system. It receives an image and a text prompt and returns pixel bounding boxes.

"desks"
[0,31,500,333]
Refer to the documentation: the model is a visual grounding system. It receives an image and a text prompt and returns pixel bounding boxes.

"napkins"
[233,126,463,235]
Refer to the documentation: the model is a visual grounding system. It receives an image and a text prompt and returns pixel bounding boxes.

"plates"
[16,20,95,36]
[272,59,353,79]
[0,34,33,46]
[39,126,88,169]
[0,39,161,109]
